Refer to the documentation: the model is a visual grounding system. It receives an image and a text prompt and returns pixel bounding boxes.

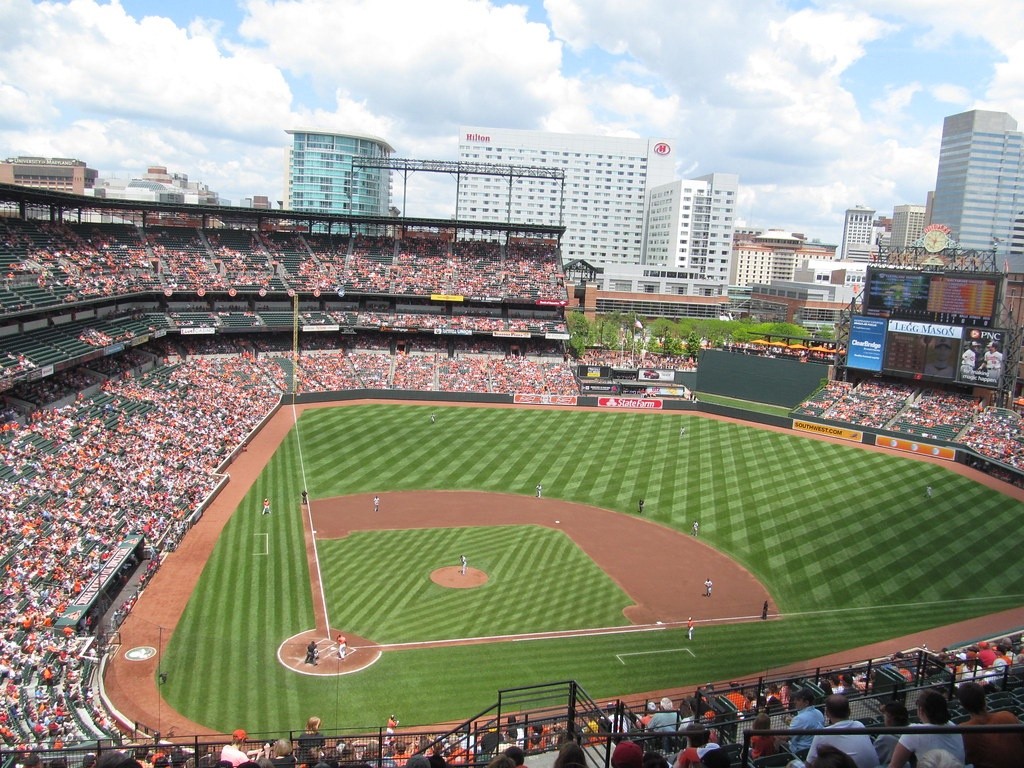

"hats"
[979,642,988,648]
[789,687,816,704]
[970,341,981,346]
[660,697,673,710]
[986,342,997,347]
[233,729,249,739]
[611,742,644,768]
[648,702,657,712]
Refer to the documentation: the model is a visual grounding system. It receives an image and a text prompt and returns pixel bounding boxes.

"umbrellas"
[770,340,786,346]
[751,338,768,345]
[831,345,845,354]
[788,343,807,350]
[809,345,830,354]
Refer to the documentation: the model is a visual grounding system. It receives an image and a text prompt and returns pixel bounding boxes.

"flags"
[634,318,644,330]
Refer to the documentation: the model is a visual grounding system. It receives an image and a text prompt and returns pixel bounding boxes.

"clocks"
[924,229,947,253]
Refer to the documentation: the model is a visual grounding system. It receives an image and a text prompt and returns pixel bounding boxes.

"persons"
[978,339,1002,381]
[372,495,381,513]
[692,519,699,536]
[535,484,542,499]
[0,489,205,768]
[688,617,693,640]
[761,600,770,621]
[961,341,981,380]
[926,486,932,498]
[459,555,467,575]
[302,490,308,505]
[220,708,603,768]
[261,498,272,516]
[431,413,435,422]
[305,641,319,667]
[704,578,713,597]
[337,635,348,660]
[602,634,1024,768]
[638,498,646,513]
[0,227,1023,489]
